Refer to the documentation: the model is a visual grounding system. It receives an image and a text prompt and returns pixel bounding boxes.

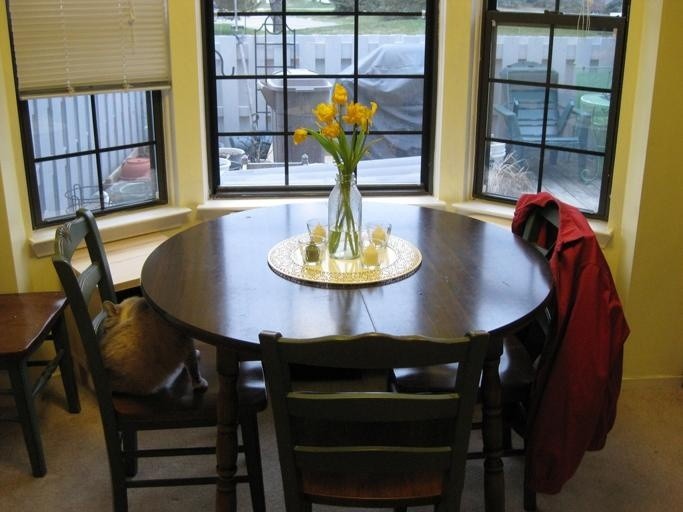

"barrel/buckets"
[267,69,333,164]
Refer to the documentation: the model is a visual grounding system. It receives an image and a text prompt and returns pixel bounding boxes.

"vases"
[121,158,150,182]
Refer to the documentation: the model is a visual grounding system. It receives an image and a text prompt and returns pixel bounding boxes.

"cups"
[301,220,329,267]
[360,222,391,271]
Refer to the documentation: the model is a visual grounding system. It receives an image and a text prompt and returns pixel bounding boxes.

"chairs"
[0,290,81,478]
[393,195,596,508]
[256,326,486,509]
[50,208,270,508]
[493,62,592,181]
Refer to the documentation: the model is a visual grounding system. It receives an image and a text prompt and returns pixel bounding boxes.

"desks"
[141,200,555,508]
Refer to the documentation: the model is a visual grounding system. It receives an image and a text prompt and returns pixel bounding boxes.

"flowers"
[291,84,384,259]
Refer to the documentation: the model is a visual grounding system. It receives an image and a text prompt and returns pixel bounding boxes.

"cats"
[98,295,210,396]
